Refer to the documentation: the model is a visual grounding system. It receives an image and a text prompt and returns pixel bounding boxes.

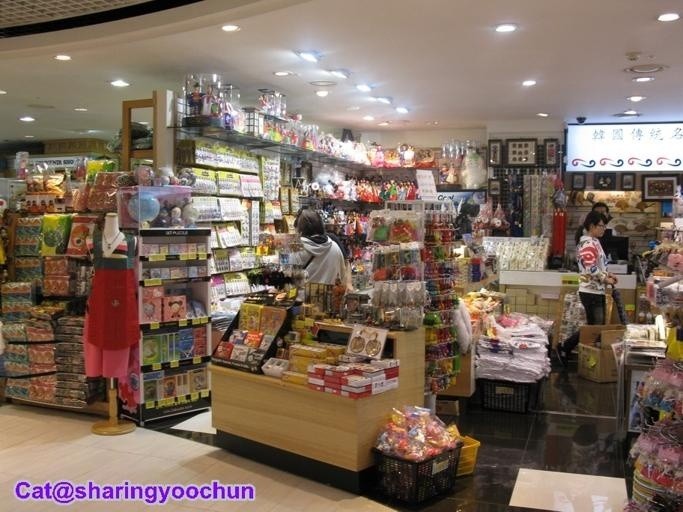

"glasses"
[597,224,607,230]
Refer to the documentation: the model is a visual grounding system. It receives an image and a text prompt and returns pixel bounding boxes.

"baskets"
[371,437,481,505]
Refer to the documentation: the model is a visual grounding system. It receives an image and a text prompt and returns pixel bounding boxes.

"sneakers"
[555,342,568,366]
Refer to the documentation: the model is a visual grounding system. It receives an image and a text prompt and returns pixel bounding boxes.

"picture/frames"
[545,139,558,165]
[506,139,538,165]
[642,174,680,202]
[621,174,636,191]
[594,173,617,189]
[572,173,587,189]
[488,139,503,167]
[488,179,501,196]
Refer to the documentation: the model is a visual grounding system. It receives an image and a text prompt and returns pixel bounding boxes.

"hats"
[295,209,324,235]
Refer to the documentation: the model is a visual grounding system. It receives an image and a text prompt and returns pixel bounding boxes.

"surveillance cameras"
[576,117,586,123]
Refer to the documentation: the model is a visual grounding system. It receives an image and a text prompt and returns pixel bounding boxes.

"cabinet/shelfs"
[178,128,481,475]
[119,186,213,429]
[0,89,176,421]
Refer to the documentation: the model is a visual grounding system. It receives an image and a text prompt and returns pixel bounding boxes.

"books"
[610,314,672,369]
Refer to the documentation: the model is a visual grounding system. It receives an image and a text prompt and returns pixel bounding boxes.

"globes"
[128,191,161,229]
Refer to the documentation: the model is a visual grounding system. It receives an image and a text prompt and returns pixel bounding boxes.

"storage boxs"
[578,325,624,384]
[373,436,461,502]
[476,380,538,416]
[464,437,479,472]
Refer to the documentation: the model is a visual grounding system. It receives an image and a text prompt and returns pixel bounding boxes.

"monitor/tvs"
[600,236,629,265]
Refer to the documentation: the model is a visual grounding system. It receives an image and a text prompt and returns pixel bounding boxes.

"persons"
[80,212,141,436]
[554,202,618,368]
[281,207,347,302]
